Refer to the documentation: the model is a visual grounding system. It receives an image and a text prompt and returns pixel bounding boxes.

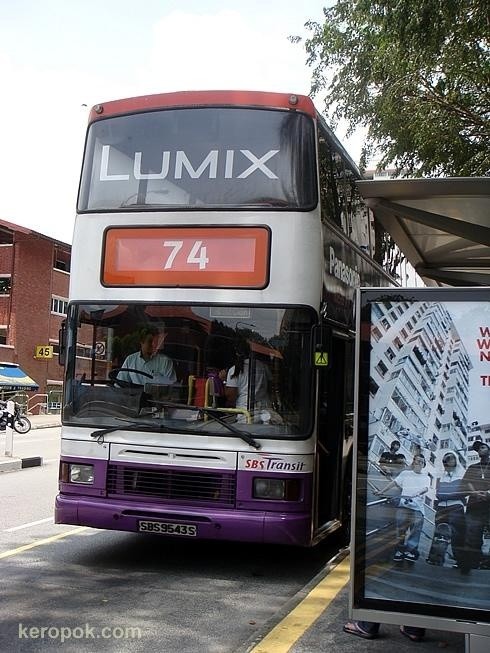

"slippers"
[401,626,421,640]
[343,623,371,639]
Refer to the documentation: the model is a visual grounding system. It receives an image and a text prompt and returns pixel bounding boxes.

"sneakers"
[393,551,403,561]
[405,552,415,563]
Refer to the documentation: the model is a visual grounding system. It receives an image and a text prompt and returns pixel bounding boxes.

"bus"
[53,89,432,564]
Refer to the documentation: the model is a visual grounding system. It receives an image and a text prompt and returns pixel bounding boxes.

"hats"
[472,441,489,449]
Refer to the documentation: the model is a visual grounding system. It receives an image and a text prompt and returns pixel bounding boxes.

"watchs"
[221,379,227,385]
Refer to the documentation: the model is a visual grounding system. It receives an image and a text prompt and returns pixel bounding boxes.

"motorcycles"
[0,399,32,434]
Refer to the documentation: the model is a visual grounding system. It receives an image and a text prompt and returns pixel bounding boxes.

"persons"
[427,447,467,568]
[378,439,402,476]
[219,332,277,430]
[369,453,431,560]
[460,438,489,576]
[108,318,179,389]
[387,454,405,475]
[341,616,428,644]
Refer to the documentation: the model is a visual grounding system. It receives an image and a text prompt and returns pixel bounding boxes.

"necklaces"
[479,461,489,480]
